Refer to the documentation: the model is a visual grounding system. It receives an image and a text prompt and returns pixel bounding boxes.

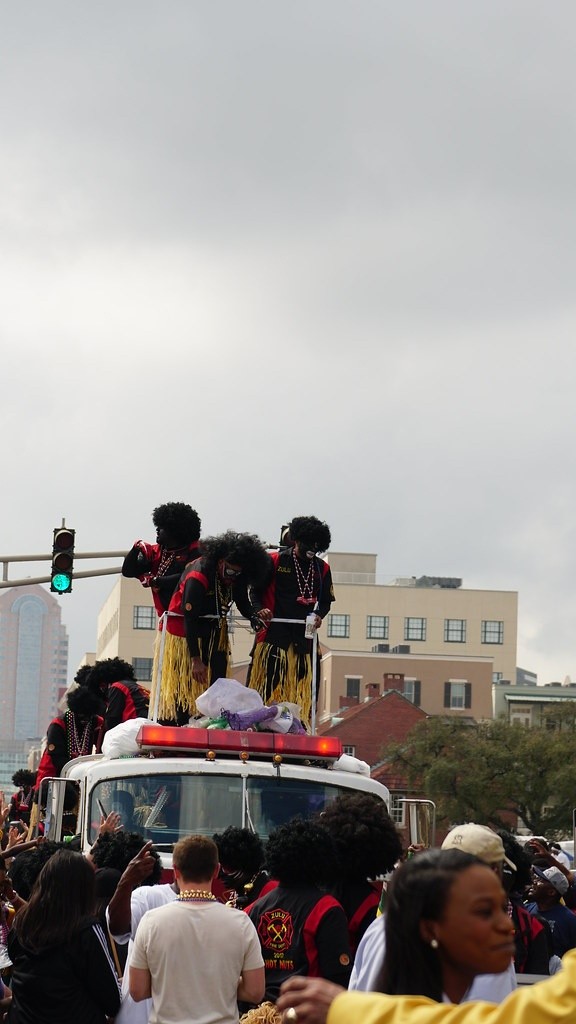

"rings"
[286,1006,298,1022]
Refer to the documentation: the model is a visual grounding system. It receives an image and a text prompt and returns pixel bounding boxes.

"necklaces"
[224,871,261,908]
[65,709,94,760]
[20,787,32,811]
[290,548,318,606]
[172,888,218,902]
[0,910,11,945]
[214,570,233,633]
[154,546,177,579]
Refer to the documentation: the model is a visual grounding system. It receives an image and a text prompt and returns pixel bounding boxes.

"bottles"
[408,850,413,860]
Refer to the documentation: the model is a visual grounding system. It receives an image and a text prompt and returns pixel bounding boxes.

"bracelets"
[5,889,20,903]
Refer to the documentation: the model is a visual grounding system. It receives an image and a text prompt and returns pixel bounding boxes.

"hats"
[530,863,570,896]
[441,823,516,871]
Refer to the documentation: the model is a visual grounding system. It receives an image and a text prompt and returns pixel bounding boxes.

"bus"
[36,725,436,853]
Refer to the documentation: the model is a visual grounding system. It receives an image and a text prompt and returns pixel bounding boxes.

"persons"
[147,531,272,730]
[122,502,201,632]
[74,656,150,754]
[25,686,106,842]
[0,768,180,1024]
[212,791,576,1024]
[128,833,265,1024]
[246,515,336,733]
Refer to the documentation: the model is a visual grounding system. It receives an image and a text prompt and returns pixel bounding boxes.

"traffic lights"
[50,528,75,594]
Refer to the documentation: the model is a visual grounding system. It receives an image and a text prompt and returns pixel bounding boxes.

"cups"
[305,616,317,639]
